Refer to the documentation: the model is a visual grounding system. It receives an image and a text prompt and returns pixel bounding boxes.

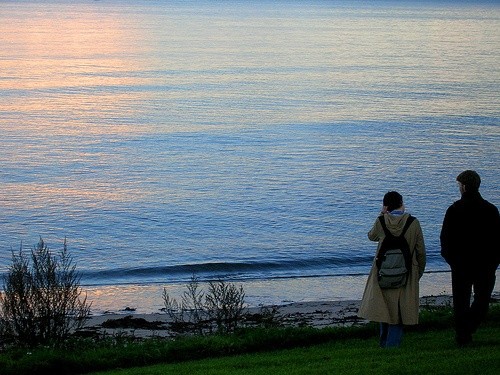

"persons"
[440,169,500,349]
[357,191,427,347]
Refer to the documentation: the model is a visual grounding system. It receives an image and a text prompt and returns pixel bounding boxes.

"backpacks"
[376,213,416,290]
